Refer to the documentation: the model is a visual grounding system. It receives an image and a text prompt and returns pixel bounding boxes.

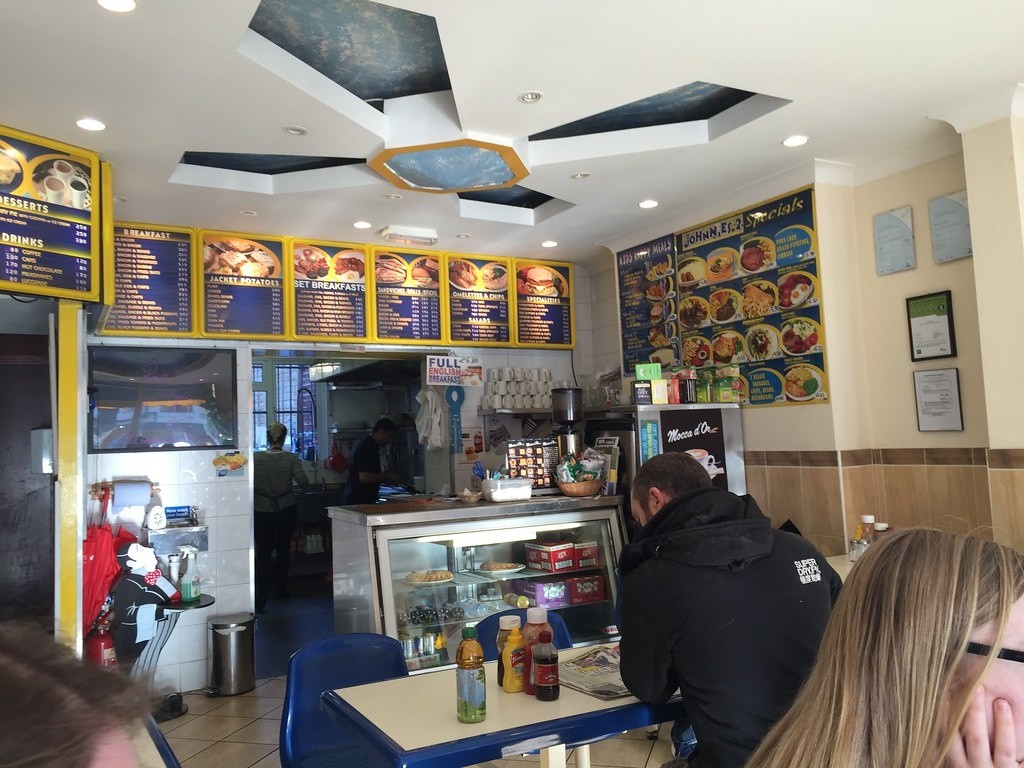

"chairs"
[475,608,627,768]
[278,631,409,768]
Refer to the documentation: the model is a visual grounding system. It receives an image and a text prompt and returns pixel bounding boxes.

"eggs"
[339,269,356,282]
[790,284,810,304]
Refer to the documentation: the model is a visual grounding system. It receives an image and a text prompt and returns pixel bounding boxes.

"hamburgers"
[411,268,430,287]
[524,268,556,294]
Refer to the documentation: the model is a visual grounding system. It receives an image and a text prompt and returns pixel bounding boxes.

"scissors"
[473,461,485,481]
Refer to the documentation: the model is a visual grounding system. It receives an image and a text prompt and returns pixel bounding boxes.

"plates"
[405,577,454,588]
[294,248,330,280]
[712,334,743,365]
[778,284,814,308]
[530,289,559,297]
[481,265,507,293]
[710,306,739,324]
[740,251,766,273]
[478,564,526,574]
[745,327,777,362]
[780,324,814,356]
[680,278,703,287]
[335,252,365,284]
[785,368,822,401]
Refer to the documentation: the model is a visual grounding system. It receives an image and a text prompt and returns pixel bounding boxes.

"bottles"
[497,616,525,693]
[456,627,486,723]
[504,593,529,609]
[523,608,553,695]
[533,631,560,701]
[298,538,303,552]
[306,534,323,553]
[449,280,478,291]
[849,515,889,563]
[397,598,488,626]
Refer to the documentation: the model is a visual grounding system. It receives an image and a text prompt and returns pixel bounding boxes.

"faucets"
[320,477,325,491]
[189,507,198,522]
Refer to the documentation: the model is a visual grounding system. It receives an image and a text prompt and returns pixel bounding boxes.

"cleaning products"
[177,544,201,601]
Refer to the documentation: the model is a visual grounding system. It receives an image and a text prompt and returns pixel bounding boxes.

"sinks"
[141,524,208,555]
[296,492,334,524]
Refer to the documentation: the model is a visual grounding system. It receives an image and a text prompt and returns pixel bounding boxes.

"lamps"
[380,225,438,246]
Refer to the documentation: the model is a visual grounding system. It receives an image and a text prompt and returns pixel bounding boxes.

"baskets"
[559,479,603,496]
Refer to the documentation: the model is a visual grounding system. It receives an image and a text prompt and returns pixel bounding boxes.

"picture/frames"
[907,289,958,362]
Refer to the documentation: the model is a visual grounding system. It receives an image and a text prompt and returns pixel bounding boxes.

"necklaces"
[271,447,283,451]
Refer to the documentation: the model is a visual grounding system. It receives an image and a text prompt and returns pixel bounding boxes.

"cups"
[481,368,570,409]
[44,160,88,209]
[615,388,632,405]
[680,378,697,403]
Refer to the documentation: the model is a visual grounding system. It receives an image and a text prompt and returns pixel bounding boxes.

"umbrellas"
[82,486,141,642]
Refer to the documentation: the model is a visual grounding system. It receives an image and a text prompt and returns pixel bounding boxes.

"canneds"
[397,631,437,659]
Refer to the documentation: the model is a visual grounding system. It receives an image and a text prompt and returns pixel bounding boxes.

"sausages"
[681,272,694,282]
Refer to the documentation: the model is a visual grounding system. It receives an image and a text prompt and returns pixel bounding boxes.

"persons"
[0,614,173,768]
[254,420,309,610]
[615,449,844,768]
[747,526,1024,768]
[343,418,403,505]
[585,646,619,664]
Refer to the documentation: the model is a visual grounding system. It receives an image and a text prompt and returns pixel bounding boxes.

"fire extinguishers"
[86,609,119,674]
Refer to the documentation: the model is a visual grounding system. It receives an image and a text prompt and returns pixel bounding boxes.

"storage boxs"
[629,362,740,404]
[513,576,571,609]
[482,478,534,503]
[594,436,621,496]
[572,540,598,569]
[524,540,576,573]
[563,573,605,605]
[405,651,440,671]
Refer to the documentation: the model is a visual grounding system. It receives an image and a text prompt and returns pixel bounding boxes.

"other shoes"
[277,591,290,599]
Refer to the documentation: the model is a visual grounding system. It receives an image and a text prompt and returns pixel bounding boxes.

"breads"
[478,560,517,569]
[406,568,454,582]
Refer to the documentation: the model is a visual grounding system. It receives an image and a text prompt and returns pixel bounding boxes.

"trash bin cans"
[206,612,256,697]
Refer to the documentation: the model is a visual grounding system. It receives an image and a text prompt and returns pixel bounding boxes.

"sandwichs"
[374,259,406,282]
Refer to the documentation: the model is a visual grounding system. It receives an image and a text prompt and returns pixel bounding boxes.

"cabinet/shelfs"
[324,494,630,677]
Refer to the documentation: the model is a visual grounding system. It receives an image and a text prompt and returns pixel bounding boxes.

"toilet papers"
[111,483,151,515]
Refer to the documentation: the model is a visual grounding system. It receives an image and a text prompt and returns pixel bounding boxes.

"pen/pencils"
[487,469,492,480]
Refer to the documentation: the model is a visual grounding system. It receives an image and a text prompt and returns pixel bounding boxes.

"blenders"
[551,388,581,465]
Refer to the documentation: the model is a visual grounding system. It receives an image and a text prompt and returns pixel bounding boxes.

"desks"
[130,593,216,724]
[319,641,687,768]
